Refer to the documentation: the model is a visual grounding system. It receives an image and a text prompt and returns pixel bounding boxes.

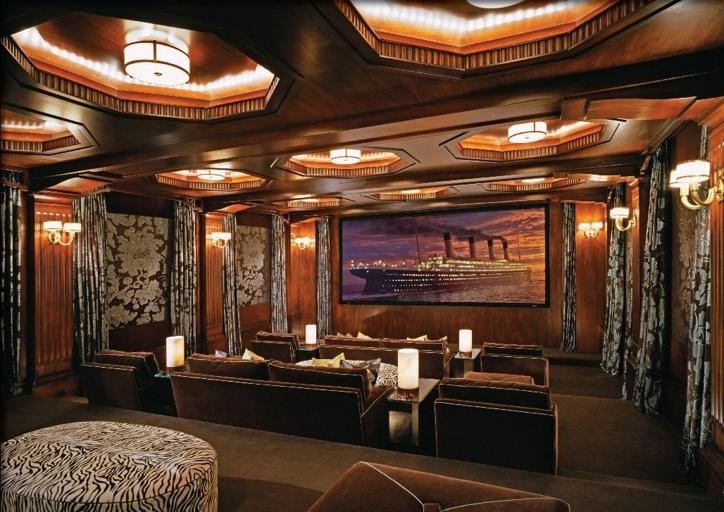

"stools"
[0,420,219,512]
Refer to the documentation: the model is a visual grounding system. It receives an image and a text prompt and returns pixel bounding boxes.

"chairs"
[79,330,558,476]
[305,460,570,512]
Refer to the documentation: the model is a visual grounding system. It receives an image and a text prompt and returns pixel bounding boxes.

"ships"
[348,224,531,296]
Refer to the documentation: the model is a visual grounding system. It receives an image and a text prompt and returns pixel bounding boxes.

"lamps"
[328,149,363,165]
[211,232,231,248]
[578,221,604,238]
[294,237,311,249]
[668,157,724,210]
[196,168,226,181]
[507,122,547,144]
[123,28,191,87]
[465,0,525,9]
[43,221,81,246]
[610,206,637,232]
